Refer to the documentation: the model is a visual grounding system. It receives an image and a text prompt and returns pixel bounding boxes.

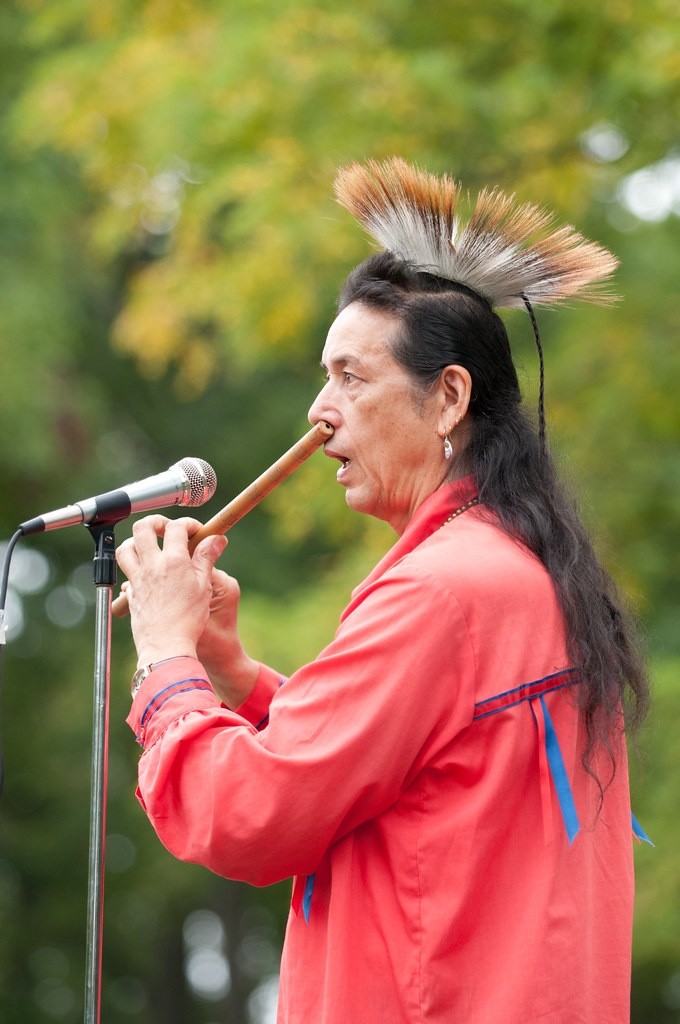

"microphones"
[16,457,217,536]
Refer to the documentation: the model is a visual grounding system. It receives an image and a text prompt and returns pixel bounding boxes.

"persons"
[116,156,654,1023]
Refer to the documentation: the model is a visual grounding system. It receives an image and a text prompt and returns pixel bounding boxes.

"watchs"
[130,655,198,701]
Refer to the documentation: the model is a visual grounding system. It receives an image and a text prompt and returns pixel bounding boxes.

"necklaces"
[439,497,486,527]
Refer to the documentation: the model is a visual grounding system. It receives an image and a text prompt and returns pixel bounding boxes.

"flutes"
[113,419,333,622]
